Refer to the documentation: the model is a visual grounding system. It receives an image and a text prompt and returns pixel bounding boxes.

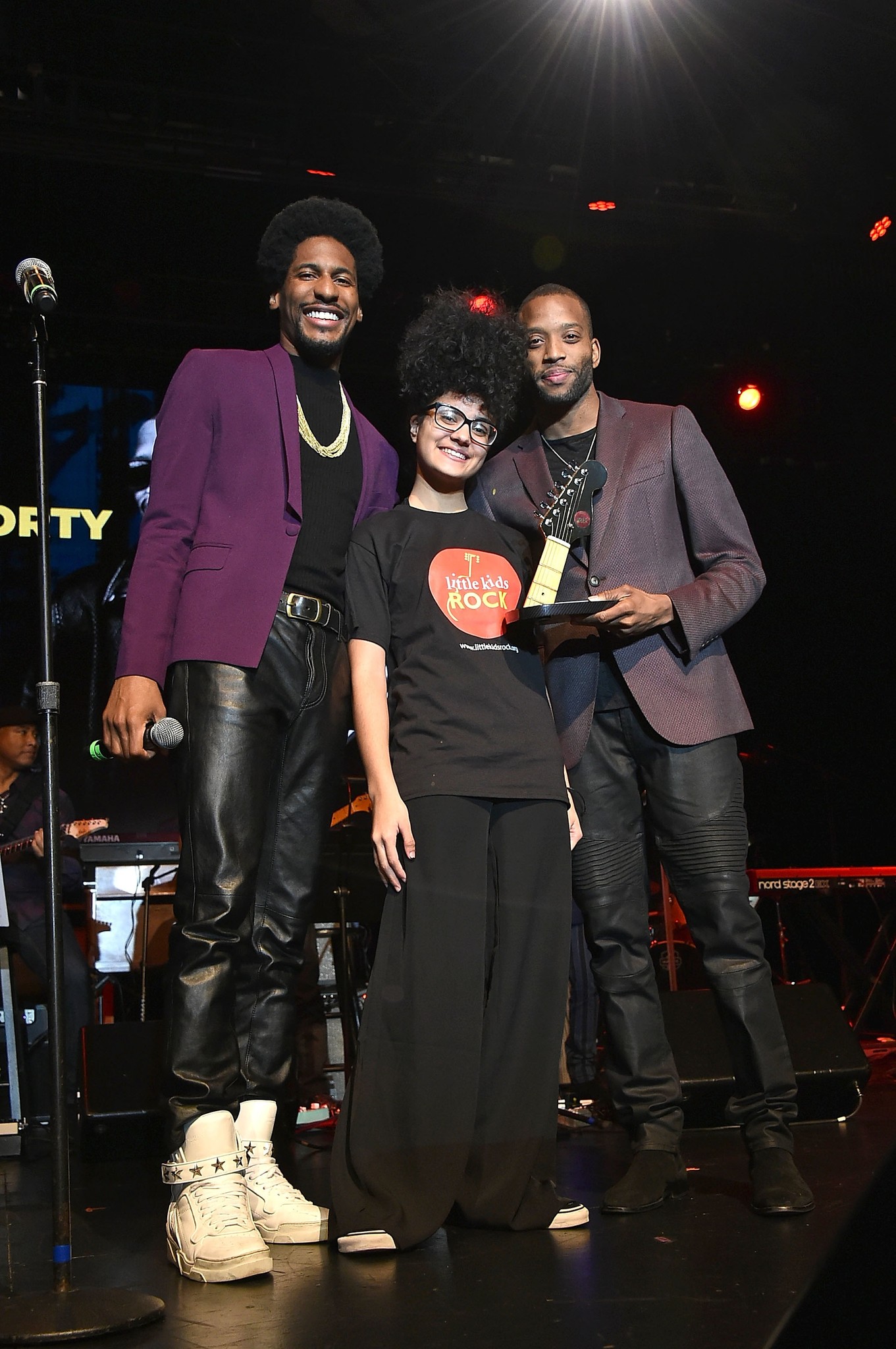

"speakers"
[76,1020,173,1122]
[322,993,346,1102]
[657,981,870,1127]
[314,921,362,987]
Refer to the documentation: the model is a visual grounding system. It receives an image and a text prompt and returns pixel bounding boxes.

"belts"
[278,590,348,635]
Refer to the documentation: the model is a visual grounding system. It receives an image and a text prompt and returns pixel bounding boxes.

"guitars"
[0,817,108,858]
[523,457,609,605]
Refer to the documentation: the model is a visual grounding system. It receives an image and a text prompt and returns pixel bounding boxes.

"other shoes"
[336,1225,397,1257]
[549,1202,591,1231]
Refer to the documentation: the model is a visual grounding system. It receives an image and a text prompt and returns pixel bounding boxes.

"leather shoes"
[603,1150,690,1213]
[748,1151,816,1215]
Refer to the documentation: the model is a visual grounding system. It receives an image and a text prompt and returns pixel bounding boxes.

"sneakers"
[159,1111,274,1283]
[230,1097,332,1243]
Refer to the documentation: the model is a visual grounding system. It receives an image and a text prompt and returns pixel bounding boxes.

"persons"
[462,281,820,1216]
[97,197,402,1291]
[1,684,120,1160]
[329,304,592,1254]
[552,765,608,1108]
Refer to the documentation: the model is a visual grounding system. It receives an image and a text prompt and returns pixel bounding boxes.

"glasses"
[414,400,499,448]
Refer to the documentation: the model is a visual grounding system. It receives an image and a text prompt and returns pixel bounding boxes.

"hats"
[0,704,42,728]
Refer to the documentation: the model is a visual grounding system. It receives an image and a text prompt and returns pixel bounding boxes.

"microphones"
[84,718,185,761]
[15,257,57,313]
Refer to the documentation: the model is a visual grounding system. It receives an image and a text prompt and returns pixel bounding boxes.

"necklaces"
[539,431,596,473]
[296,378,355,461]
